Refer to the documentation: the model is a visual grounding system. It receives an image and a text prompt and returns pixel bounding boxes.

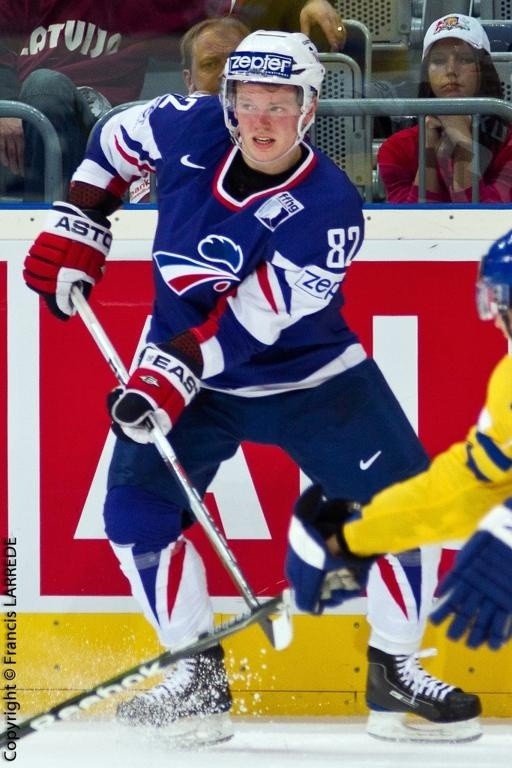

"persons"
[281,229,511,654]
[127,16,251,199]
[1,0,349,197]
[237,0,335,53]
[23,26,484,727]
[376,11,512,203]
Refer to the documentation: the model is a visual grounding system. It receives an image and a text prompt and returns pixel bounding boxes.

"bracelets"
[335,522,351,558]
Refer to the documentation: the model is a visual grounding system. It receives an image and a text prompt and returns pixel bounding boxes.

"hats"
[421,14,491,58]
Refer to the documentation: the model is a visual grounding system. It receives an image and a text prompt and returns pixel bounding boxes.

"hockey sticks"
[68,286,293,654]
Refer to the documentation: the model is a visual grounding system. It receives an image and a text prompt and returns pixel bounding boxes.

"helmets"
[218,30,326,99]
[479,230,512,285]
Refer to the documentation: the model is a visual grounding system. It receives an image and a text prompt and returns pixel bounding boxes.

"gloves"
[285,485,380,614]
[431,505,511,648]
[107,344,202,442]
[22,199,112,320]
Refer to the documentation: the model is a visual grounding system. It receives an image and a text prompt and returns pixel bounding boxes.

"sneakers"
[115,651,233,726]
[367,645,482,722]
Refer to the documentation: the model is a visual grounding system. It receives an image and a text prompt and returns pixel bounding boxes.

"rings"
[337,25,344,32]
